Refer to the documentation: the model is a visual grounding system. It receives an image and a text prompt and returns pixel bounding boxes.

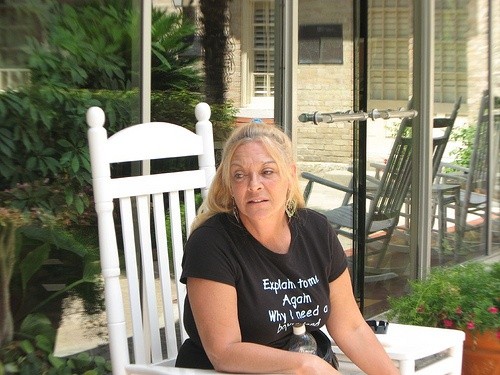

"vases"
[459,325,500,375]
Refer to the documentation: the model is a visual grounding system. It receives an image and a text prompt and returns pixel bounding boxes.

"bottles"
[288,322,318,355]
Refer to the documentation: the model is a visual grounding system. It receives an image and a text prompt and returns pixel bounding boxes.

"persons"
[175,123,401,375]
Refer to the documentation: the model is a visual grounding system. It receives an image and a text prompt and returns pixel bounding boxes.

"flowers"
[387,260,500,339]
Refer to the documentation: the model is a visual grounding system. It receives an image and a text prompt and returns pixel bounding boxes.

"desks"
[432,184,462,262]
[318,321,466,375]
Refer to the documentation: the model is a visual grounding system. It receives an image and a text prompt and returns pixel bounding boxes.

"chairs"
[301,97,410,284]
[87,102,252,375]
[431,89,500,264]
[367,97,460,252]
